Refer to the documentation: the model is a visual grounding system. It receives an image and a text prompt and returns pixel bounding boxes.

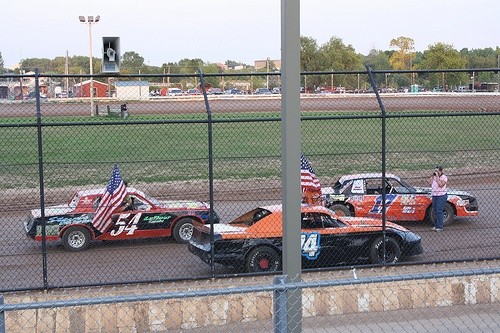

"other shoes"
[435,229,444,232]
[432,227,436,230]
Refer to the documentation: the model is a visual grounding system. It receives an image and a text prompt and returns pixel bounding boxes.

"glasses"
[377,183,381,185]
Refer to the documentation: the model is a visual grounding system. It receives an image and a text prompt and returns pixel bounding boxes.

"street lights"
[79,15,100,116]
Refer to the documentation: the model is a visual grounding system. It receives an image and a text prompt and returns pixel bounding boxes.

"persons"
[431,167,448,232]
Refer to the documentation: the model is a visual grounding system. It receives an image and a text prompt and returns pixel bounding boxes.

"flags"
[91,164,132,234]
[301,154,321,192]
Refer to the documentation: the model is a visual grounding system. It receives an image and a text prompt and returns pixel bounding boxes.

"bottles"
[433,172,439,176]
[353,267,357,279]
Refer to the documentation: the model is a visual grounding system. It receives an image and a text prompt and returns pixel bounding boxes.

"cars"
[150,88,280,96]
[321,173,479,228]
[22,187,220,253]
[187,204,424,273]
[300,86,468,93]
